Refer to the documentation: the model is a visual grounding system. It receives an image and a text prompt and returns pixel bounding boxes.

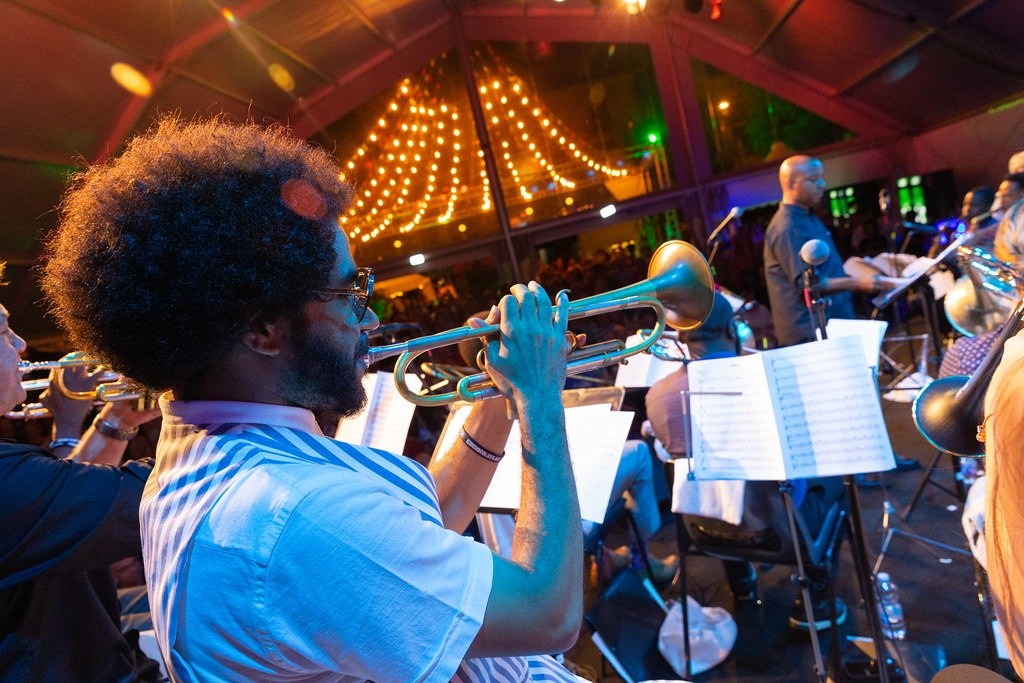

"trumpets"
[912,294,1024,458]
[17,350,159,414]
[363,240,715,408]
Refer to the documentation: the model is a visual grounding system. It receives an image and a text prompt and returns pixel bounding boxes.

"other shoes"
[894,453,920,471]
[855,477,894,488]
[590,542,628,587]
[631,551,678,591]
[789,599,847,630]
[731,575,759,614]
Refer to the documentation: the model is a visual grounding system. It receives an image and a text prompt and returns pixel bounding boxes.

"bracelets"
[458,426,506,462]
[49,437,80,449]
[93,414,141,441]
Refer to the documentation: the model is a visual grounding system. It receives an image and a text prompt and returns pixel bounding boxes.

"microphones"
[800,239,831,314]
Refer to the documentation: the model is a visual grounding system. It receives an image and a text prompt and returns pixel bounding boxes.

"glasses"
[296,267,374,322]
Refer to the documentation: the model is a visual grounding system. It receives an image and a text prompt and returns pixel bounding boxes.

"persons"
[459,312,680,584]
[766,154,922,487]
[926,150,1024,683]
[40,114,593,683]
[0,260,163,683]
[642,290,847,631]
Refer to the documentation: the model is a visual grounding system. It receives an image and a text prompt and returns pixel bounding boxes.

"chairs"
[464,474,869,683]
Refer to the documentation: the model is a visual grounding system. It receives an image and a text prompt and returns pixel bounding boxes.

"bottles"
[873,572,906,639]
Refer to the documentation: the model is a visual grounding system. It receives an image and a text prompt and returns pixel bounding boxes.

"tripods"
[896,274,984,524]
